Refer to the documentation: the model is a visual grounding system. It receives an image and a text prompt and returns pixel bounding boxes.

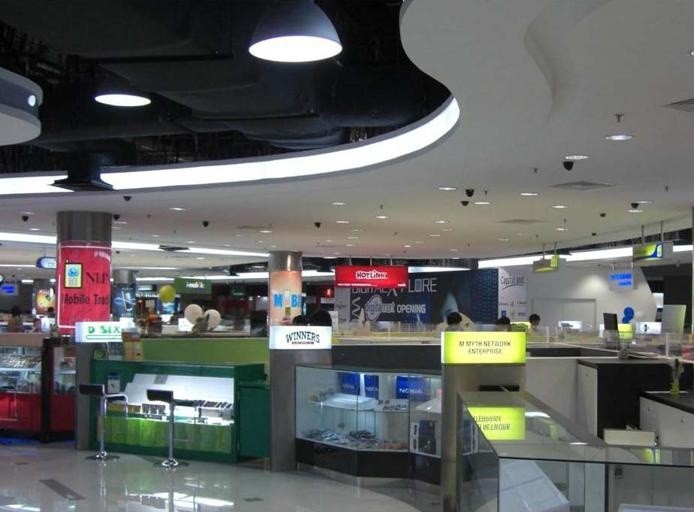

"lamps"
[92,70,152,107]
[247,0,344,63]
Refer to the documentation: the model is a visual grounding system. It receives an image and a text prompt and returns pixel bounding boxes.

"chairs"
[79,383,128,459]
[147,389,194,467]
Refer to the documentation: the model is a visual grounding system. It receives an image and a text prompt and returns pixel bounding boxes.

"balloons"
[159,285,175,302]
[185,304,204,325]
[203,309,221,329]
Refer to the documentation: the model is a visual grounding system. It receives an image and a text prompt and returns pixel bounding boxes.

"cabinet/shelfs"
[575,365,673,441]
[89,359,269,463]
[1,333,77,443]
[458,391,693,512]
[295,365,442,486]
[640,394,693,449]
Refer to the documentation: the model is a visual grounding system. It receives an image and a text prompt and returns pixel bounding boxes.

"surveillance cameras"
[465,189,474,197]
[20,215,31,223]
[123,196,132,201]
[600,213,606,218]
[460,201,469,206]
[113,215,120,220]
[592,233,596,236]
[203,221,208,227]
[563,160,574,170]
[314,222,321,227]
[630,203,639,209]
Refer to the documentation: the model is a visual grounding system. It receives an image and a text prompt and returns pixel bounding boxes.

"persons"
[495,317,512,332]
[293,315,309,326]
[0,303,57,332]
[307,302,332,326]
[444,311,464,331]
[525,314,542,336]
